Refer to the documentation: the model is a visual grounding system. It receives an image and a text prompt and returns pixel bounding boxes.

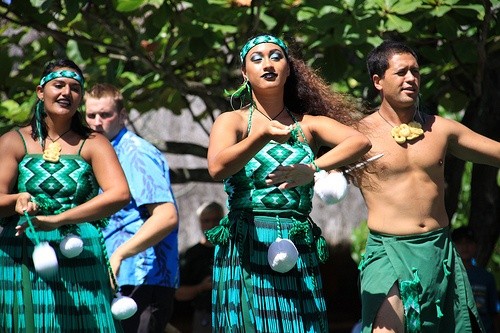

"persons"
[316,40,500,333]
[173,201,226,333]
[452,225,500,333]
[203,32,395,333]
[0,57,138,333]
[83,82,180,333]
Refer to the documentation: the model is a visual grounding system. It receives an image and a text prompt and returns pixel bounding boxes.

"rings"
[285,176,288,181]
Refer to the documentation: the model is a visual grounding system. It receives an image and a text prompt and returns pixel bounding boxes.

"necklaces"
[253,105,285,121]
[378,109,424,144]
[43,127,72,162]
